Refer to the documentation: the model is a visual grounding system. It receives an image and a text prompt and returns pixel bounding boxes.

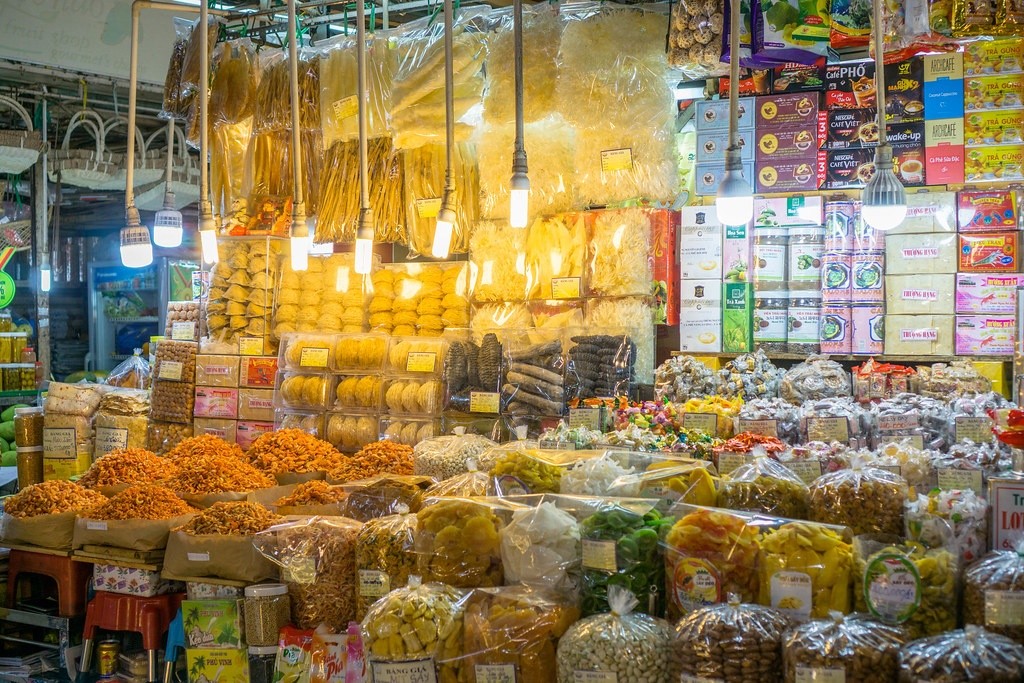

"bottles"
[0,313,42,492]
[243,585,290,647]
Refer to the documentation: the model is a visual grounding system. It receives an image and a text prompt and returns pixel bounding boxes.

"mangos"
[0,403,31,467]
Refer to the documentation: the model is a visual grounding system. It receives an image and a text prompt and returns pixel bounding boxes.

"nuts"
[553,605,1024,683]
[579,505,675,616]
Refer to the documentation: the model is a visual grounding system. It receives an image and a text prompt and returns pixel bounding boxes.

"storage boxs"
[754,195,825,227]
[695,44,1024,196]
[883,191,958,357]
[93,563,187,597]
[972,362,1011,398]
[956,273,1023,355]
[681,205,754,354]
[182,581,250,683]
[193,355,285,451]
[469,208,681,326]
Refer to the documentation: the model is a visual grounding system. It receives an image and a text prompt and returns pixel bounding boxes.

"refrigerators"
[84,258,198,372]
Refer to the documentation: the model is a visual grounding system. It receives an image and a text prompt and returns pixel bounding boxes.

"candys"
[548,393,1016,486]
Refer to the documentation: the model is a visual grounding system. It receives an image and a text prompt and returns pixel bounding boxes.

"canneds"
[13,407,43,488]
[0,313,45,392]
[244,584,290,683]
[95,639,120,678]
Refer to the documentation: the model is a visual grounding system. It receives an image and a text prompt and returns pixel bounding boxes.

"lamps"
[676,78,720,100]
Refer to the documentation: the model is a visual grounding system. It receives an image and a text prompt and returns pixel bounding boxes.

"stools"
[79,590,187,683]
[0,548,93,678]
[164,607,188,683]
